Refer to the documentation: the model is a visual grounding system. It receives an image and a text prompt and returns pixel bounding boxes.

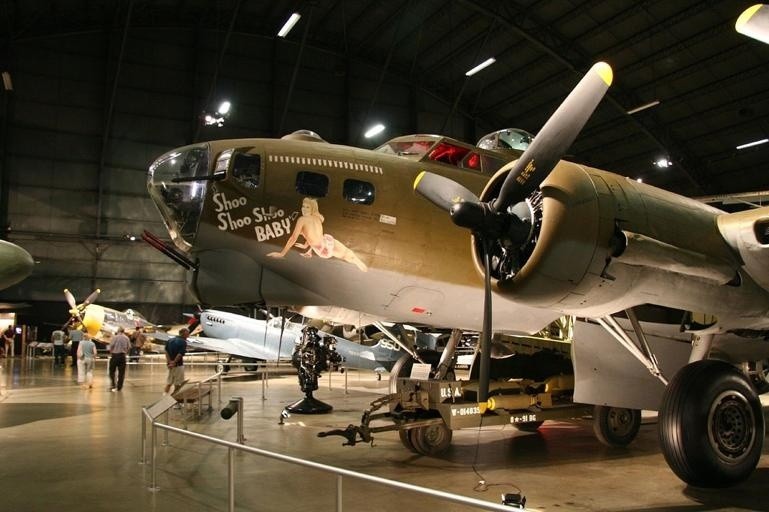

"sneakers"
[109,384,116,390]
[116,386,122,391]
[163,392,168,396]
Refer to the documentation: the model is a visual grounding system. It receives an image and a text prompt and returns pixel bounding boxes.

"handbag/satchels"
[78,350,85,360]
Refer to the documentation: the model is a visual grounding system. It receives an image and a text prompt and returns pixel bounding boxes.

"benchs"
[170,383,216,422]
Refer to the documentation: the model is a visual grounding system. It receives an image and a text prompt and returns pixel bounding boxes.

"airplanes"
[54,285,203,355]
[143,54,768,496]
[139,302,417,380]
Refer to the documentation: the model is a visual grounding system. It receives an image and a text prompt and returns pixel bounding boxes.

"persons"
[51,324,67,365]
[108,326,131,393]
[163,328,190,409]
[129,327,141,362]
[264,193,369,273]
[3,325,16,357]
[76,333,98,389]
[64,324,85,366]
